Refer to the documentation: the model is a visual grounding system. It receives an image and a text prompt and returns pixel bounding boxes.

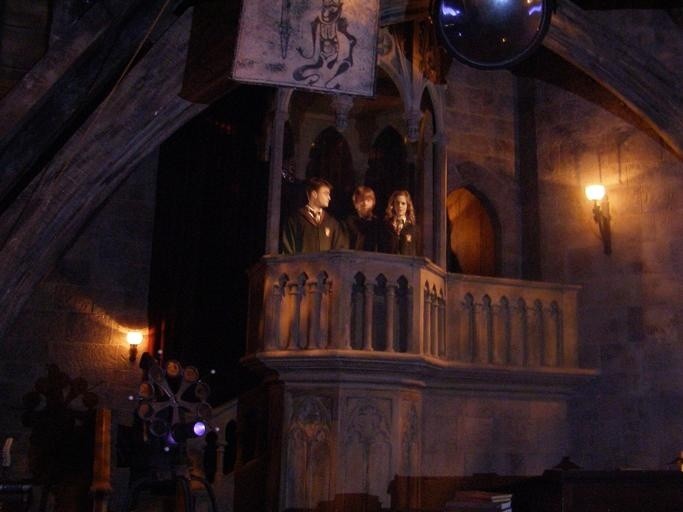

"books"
[445,489,514,512]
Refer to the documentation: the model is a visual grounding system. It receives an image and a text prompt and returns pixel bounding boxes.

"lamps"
[127,332,144,361]
[584,183,614,255]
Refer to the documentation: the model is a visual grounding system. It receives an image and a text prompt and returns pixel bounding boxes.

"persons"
[279,176,347,251]
[340,185,381,254]
[374,189,417,256]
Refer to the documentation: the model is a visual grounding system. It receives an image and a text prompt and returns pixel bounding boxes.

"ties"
[396,219,403,236]
[310,210,320,223]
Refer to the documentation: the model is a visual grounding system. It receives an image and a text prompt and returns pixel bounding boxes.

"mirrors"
[434,1,550,69]
